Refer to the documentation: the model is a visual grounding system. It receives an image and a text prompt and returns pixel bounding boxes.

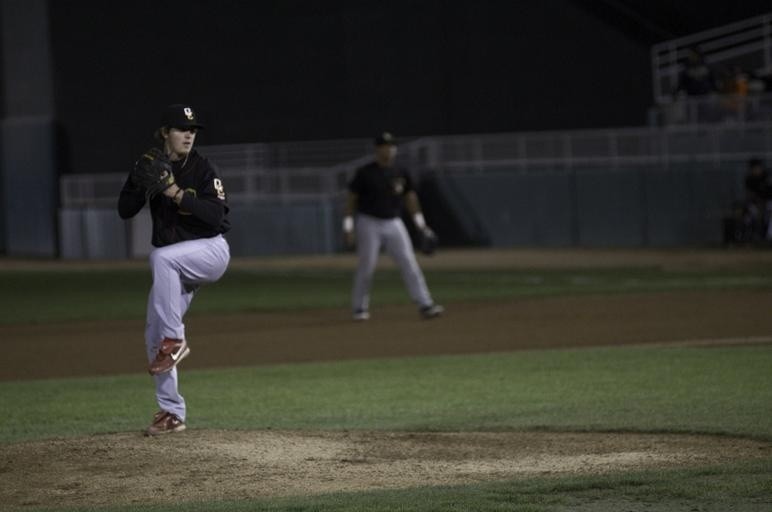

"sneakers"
[148,338,190,376]
[352,310,370,321]
[420,301,442,320]
[144,409,185,437]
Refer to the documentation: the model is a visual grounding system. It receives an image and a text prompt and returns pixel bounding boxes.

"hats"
[160,105,202,130]
[373,132,402,145]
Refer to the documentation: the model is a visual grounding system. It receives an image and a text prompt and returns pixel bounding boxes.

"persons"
[117,100,231,436]
[342,132,445,320]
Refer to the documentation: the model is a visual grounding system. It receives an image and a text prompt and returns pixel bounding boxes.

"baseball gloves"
[417,226,438,256]
[139,145,176,193]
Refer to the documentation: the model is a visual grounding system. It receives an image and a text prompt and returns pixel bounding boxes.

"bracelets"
[171,188,182,202]
[343,217,354,233]
[413,211,426,230]
